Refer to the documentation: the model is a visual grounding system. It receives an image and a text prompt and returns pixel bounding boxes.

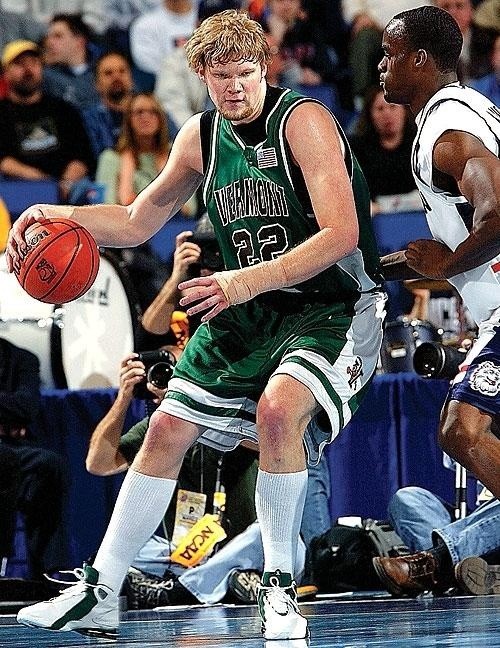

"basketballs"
[11,217,100,304]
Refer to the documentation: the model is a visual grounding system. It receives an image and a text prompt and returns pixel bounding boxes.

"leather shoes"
[453,556,500,597]
[372,541,452,600]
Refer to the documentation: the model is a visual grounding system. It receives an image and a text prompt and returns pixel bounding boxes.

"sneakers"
[13,560,124,642]
[227,568,261,605]
[125,572,174,607]
[256,572,312,644]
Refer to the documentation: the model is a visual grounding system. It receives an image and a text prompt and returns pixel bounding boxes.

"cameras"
[412,342,492,381]
[132,351,176,399]
[188,234,222,272]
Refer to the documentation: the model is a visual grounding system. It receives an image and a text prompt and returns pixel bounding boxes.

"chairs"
[0,24,500,327]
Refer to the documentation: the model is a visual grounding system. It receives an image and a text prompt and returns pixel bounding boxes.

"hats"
[1,39,41,68]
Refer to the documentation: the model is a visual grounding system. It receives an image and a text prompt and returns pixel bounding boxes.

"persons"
[298,448,335,549]
[1,5,395,642]
[377,5,500,508]
[371,478,500,605]
[83,340,307,608]
[1,337,73,580]
